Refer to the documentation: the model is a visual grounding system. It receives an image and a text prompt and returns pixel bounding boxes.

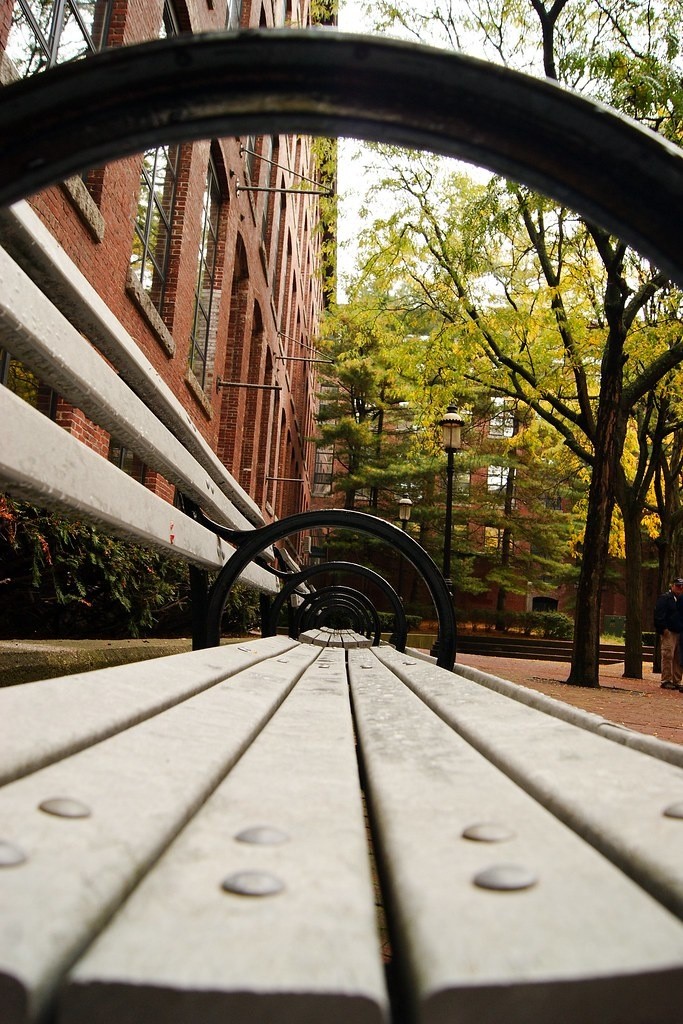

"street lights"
[388,492,414,644]
[434,405,467,657]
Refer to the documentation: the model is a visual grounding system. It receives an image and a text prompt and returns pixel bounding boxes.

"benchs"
[8,23,683,1024]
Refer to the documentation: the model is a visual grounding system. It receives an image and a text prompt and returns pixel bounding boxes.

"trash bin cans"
[603,614,624,638]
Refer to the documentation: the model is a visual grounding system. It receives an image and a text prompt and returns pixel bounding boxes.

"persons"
[654,578,682,690]
